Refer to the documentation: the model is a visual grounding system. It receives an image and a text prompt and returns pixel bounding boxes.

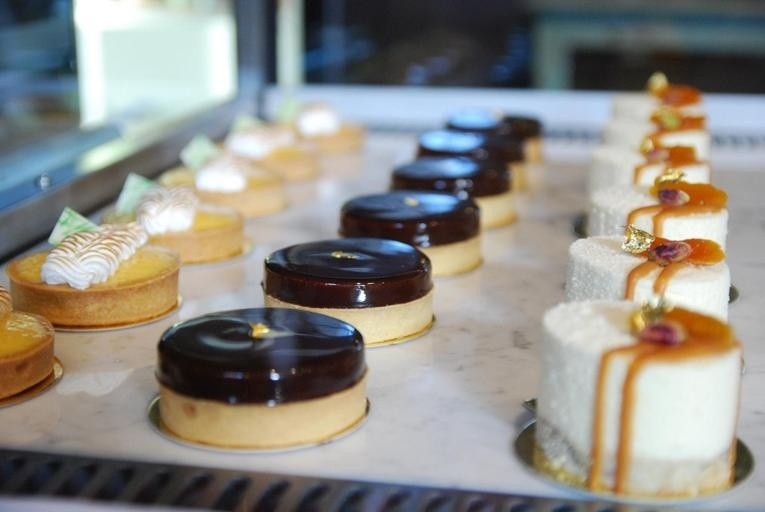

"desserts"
[388,107,547,228]
[590,71,709,228]
[193,99,364,216]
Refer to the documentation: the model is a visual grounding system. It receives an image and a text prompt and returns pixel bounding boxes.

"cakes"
[583,170,729,257]
[532,293,743,501]
[6,204,179,329]
[261,238,434,344]
[339,190,481,277]
[1,286,54,400]
[563,224,732,323]
[154,307,369,446]
[98,171,244,266]
